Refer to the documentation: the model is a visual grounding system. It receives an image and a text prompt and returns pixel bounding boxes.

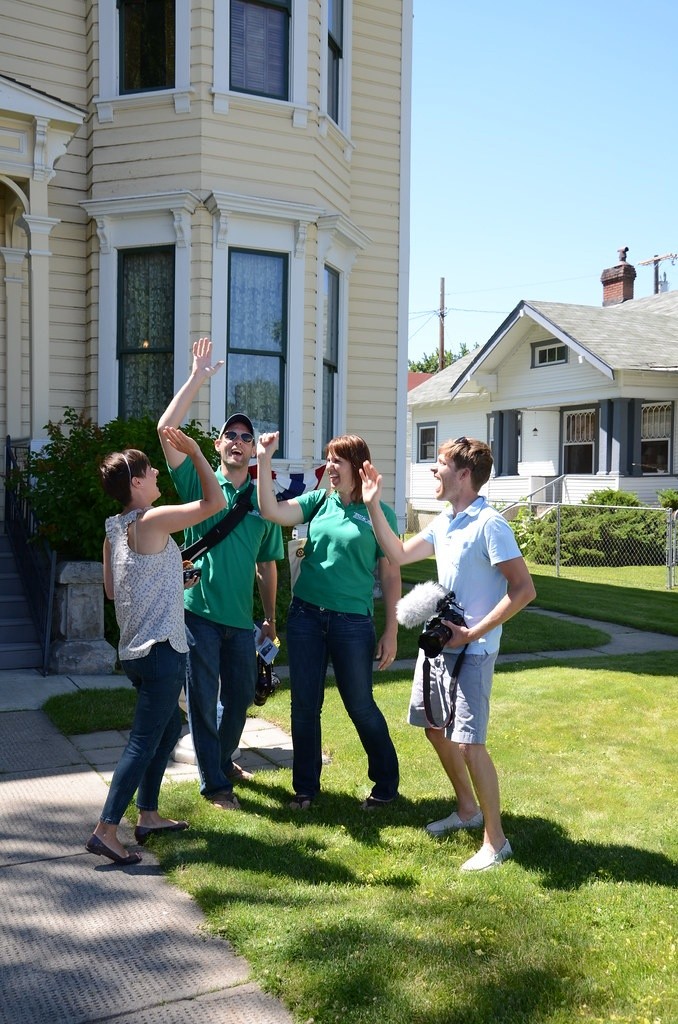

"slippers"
[231,761,243,779]
[365,792,399,810]
[288,796,311,810]
[209,793,235,805]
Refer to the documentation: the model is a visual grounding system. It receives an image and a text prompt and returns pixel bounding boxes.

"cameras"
[418,609,463,658]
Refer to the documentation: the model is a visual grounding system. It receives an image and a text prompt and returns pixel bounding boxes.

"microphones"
[395,581,445,628]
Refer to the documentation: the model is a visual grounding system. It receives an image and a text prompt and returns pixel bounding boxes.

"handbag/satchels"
[288,492,326,606]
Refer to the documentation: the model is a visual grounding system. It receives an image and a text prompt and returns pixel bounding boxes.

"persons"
[358,437,540,873]
[252,432,403,812]
[158,337,285,817]
[85,424,228,865]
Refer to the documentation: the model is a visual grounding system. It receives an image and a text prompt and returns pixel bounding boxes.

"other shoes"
[86,834,143,864]
[459,838,513,872]
[425,806,483,834]
[134,819,190,845]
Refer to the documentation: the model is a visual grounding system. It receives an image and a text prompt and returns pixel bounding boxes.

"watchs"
[263,617,276,623]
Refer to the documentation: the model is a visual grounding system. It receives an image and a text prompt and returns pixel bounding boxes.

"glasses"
[221,431,255,445]
[454,436,471,450]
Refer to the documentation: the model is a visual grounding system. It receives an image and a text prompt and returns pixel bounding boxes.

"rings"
[390,657,394,663]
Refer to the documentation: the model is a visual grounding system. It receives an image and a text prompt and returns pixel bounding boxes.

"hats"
[218,413,255,446]
